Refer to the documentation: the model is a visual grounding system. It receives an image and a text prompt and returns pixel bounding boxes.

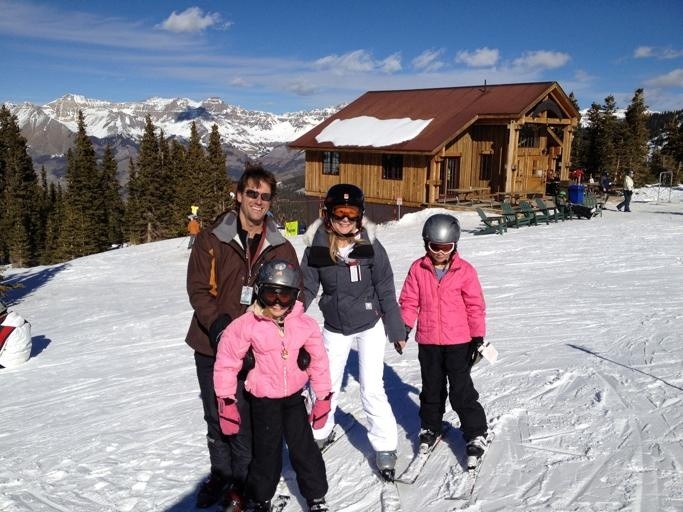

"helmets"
[254,260,301,303]
[423,215,460,251]
[324,184,365,221]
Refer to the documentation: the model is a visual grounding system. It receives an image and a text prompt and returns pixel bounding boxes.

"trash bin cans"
[568,184,585,204]
[558,180,575,203]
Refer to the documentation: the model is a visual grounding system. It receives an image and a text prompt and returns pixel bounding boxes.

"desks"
[447,187,488,206]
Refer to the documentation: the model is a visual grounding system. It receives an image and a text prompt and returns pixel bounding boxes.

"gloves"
[217,396,241,436]
[210,315,255,380]
[297,350,310,369]
[308,395,333,429]
[394,326,410,355]
[467,338,483,366]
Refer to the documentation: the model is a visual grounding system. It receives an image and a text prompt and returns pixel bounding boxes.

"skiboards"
[269,494,328,511]
[394,414,498,500]
[314,413,401,512]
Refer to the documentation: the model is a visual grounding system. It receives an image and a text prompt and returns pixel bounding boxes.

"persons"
[398,215,485,460]
[616,171,634,212]
[587,172,596,183]
[267,210,273,217]
[187,216,201,249]
[214,259,337,511]
[0,301,32,369]
[189,203,200,220]
[600,171,613,209]
[184,167,307,511]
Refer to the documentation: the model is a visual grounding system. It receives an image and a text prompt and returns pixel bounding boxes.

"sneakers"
[197,429,488,512]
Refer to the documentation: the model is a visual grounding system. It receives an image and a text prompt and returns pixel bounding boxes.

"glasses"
[330,206,361,221]
[428,241,455,255]
[259,286,297,307]
[246,189,272,201]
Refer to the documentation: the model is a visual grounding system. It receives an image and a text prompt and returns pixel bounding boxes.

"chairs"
[501,201,530,228]
[535,198,564,223]
[475,207,507,235]
[518,200,549,226]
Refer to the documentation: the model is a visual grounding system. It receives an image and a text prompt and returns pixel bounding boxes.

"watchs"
[297,184,405,470]
